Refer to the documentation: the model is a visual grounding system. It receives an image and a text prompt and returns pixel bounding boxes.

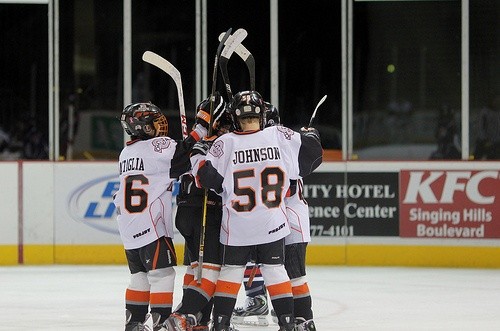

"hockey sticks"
[196,28,256,283]
[247,95,327,288]
[142,51,188,140]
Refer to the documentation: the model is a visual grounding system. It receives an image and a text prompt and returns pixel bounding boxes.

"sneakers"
[271,309,278,324]
[230,294,270,327]
[164,312,197,331]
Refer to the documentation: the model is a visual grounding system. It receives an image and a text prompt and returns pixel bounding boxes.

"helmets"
[196,96,233,137]
[229,90,265,130]
[121,102,168,140]
[263,101,280,128]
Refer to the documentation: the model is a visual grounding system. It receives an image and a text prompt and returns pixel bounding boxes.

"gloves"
[191,135,219,156]
[196,91,227,134]
[299,127,320,142]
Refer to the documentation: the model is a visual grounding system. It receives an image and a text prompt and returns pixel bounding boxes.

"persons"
[162,95,223,331]
[233,101,316,331]
[388,91,500,160]
[115,96,226,331]
[190,91,322,331]
[0,131,50,160]
